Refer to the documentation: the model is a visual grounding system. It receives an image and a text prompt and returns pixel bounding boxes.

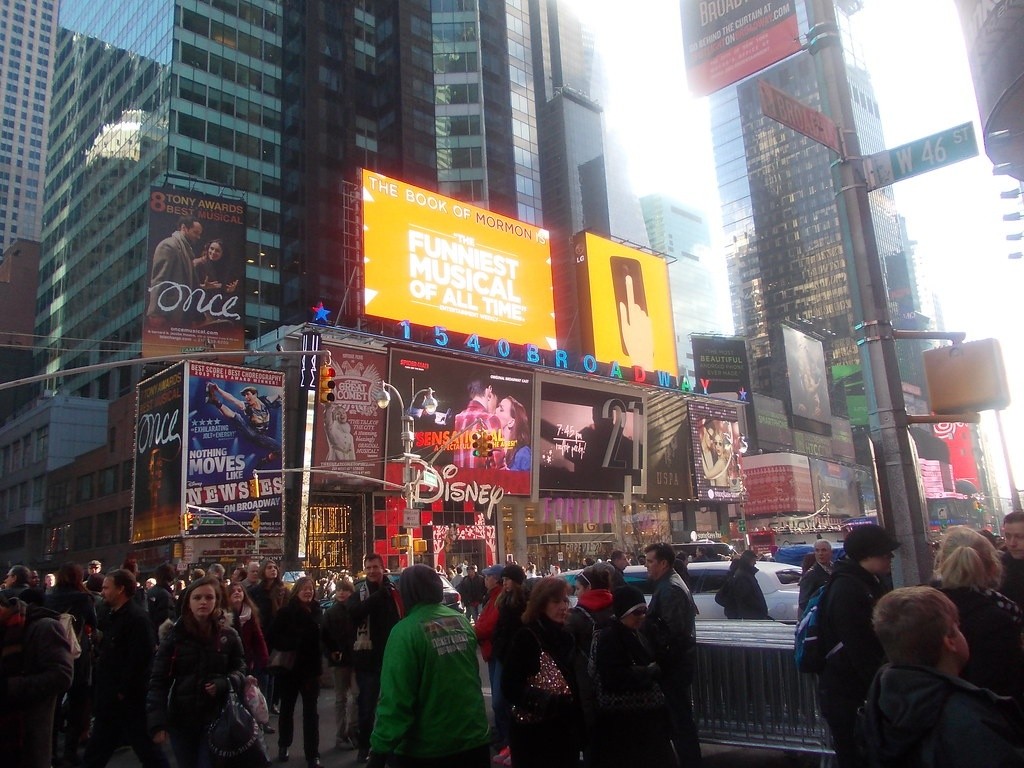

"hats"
[741,550,758,559]
[482,564,504,576]
[88,560,101,565]
[615,585,647,619]
[501,565,525,585]
[843,523,900,562]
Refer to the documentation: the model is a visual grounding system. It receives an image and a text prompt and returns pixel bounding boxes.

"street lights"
[377,380,438,565]
[821,492,831,525]
[733,453,749,550]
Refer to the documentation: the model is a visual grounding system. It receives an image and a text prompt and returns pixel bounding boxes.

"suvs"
[325,572,466,615]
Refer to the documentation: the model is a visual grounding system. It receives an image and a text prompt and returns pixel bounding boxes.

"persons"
[0,543,773,767]
[204,381,282,468]
[147,213,240,317]
[792,511,1024,768]
[698,421,730,487]
[452,379,533,470]
[324,402,357,461]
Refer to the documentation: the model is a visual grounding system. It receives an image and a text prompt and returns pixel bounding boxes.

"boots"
[205,382,218,404]
[256,456,269,470]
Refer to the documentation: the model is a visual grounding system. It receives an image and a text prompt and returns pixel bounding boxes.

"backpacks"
[575,605,602,678]
[794,572,873,674]
[57,605,85,660]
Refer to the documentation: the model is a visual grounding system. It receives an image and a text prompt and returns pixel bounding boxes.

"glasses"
[578,571,591,586]
[632,610,647,616]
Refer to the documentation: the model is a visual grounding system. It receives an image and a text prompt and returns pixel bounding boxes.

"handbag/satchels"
[206,672,270,758]
[506,626,572,724]
[266,651,297,676]
[593,660,665,713]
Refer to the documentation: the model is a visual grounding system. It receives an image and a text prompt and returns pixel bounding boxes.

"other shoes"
[358,754,369,763]
[271,708,280,714]
[492,745,511,766]
[64,752,82,766]
[336,736,360,750]
[263,724,276,733]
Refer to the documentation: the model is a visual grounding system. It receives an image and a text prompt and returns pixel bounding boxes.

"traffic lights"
[178,513,186,531]
[472,432,482,457]
[319,365,336,404]
[186,514,194,531]
[482,435,494,458]
[391,535,411,548]
[251,518,260,529]
[250,479,259,497]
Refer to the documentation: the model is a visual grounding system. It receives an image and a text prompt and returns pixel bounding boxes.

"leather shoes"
[308,756,320,768]
[279,746,289,761]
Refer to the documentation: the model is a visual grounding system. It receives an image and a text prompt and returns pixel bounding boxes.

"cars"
[551,540,802,623]
[281,571,306,590]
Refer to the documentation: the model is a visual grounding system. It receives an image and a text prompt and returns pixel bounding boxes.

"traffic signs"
[760,80,841,154]
[862,119,981,191]
